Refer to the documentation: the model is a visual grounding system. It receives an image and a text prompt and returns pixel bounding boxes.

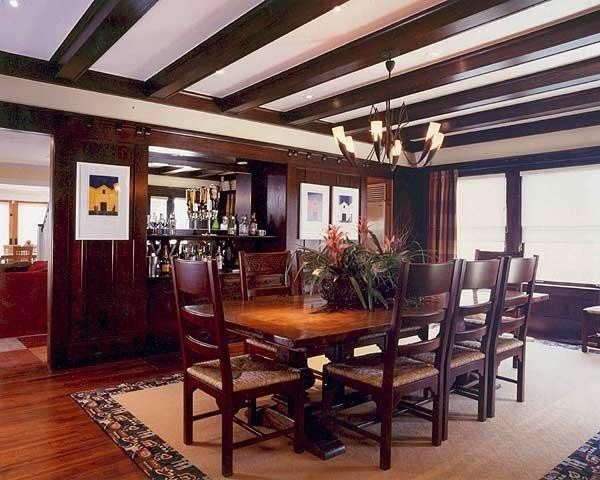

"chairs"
[320,259,461,472]
[296,249,321,295]
[474,249,526,260]
[238,251,336,364]
[401,255,504,442]
[169,254,306,476]
[455,254,539,418]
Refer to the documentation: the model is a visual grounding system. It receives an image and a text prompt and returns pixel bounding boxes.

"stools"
[580,306,600,352]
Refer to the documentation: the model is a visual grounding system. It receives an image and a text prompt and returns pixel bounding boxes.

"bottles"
[170,238,178,258]
[158,215,165,235]
[14,239,18,247]
[206,243,212,260]
[180,240,189,259]
[189,246,195,261]
[169,215,176,234]
[146,240,156,279]
[250,215,257,235]
[22,240,31,247]
[228,219,236,236]
[148,214,153,234]
[215,246,223,273]
[226,247,233,267]
[156,241,161,278]
[198,242,204,259]
[220,216,227,229]
[160,245,170,279]
[100,190,108,215]
[239,217,248,235]
[213,218,219,231]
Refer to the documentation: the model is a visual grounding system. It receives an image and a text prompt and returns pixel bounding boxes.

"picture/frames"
[299,182,329,242]
[331,185,360,241]
[75,162,129,240]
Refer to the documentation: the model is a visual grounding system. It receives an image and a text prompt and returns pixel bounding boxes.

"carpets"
[70,324,600,480]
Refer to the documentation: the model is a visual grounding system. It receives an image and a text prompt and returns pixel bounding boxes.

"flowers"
[285,224,429,311]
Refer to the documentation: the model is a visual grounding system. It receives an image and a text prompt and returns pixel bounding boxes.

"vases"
[318,276,370,309]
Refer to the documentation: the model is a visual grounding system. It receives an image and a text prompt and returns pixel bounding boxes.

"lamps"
[332,101,445,174]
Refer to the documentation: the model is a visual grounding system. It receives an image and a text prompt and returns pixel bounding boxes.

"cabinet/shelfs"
[148,228,278,353]
